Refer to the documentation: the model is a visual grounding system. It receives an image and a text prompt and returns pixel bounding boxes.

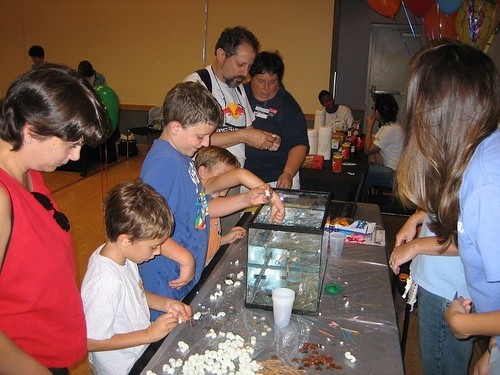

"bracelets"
[365,129,373,132]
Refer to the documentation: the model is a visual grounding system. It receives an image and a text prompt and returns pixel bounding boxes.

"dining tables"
[295,134,371,204]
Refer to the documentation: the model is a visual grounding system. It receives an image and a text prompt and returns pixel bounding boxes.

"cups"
[329,232,345,257]
[272,288,295,327]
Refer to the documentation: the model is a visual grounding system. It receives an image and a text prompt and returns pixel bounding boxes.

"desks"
[126,196,406,375]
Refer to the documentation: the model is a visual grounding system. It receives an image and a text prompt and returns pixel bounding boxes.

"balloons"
[367,0,500,51]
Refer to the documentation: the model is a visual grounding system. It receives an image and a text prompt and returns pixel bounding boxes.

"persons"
[387,38,478,375]
[0,65,112,375]
[363,90,409,190]
[79,176,192,375]
[138,80,273,326]
[28,45,49,68]
[240,50,311,216]
[407,43,500,375]
[78,60,107,88]
[195,145,286,268]
[318,90,356,131]
[181,24,282,238]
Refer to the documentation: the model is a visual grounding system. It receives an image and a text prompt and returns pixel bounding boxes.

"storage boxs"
[244,186,334,315]
